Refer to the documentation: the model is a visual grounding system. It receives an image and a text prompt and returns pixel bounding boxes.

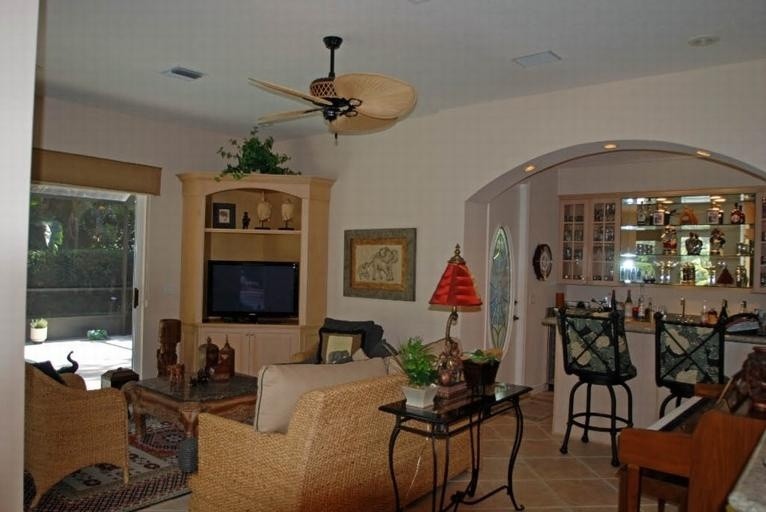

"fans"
[241,37,420,144]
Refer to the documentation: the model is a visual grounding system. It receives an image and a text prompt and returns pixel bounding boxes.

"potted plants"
[464,346,501,385]
[394,337,441,408]
[29,317,49,340]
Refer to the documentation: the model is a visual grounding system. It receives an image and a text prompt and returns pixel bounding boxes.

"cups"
[556,292,565,307]
[565,214,583,279]
[170,365,185,387]
[545,307,555,320]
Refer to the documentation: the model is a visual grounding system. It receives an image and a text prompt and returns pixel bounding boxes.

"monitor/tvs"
[207,260,299,324]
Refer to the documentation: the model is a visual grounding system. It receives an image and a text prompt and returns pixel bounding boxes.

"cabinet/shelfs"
[621,185,756,291]
[173,169,338,378]
[754,186,765,294]
[558,192,620,286]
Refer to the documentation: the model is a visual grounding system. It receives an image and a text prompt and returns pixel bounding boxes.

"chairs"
[654,309,734,420]
[554,304,640,467]
[291,317,386,368]
[23,358,130,511]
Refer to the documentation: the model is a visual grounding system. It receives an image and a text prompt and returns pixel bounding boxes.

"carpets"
[24,403,193,511]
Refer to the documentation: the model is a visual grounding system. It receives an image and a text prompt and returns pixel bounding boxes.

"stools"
[613,463,689,512]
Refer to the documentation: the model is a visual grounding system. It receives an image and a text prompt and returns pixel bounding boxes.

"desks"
[377,381,535,511]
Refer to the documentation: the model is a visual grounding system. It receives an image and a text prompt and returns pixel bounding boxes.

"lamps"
[425,243,484,399]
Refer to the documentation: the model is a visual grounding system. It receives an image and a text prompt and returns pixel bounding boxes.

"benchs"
[184,335,483,511]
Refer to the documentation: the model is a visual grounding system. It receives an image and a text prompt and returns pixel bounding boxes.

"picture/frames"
[342,225,419,303]
[213,202,237,229]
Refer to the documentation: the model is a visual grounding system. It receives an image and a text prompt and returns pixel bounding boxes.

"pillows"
[314,318,383,364]
[29,361,68,385]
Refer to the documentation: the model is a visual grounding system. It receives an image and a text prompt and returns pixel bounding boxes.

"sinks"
[664,313,702,324]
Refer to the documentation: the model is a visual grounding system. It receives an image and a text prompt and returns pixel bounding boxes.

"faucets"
[680,295,686,317]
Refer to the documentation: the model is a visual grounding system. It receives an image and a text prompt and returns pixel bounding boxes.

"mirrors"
[636,202,752,286]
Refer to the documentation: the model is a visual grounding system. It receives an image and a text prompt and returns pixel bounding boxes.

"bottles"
[592,203,614,281]
[198,336,218,369]
[217,342,236,377]
[611,288,761,333]
[621,198,750,286]
[759,194,765,287]
